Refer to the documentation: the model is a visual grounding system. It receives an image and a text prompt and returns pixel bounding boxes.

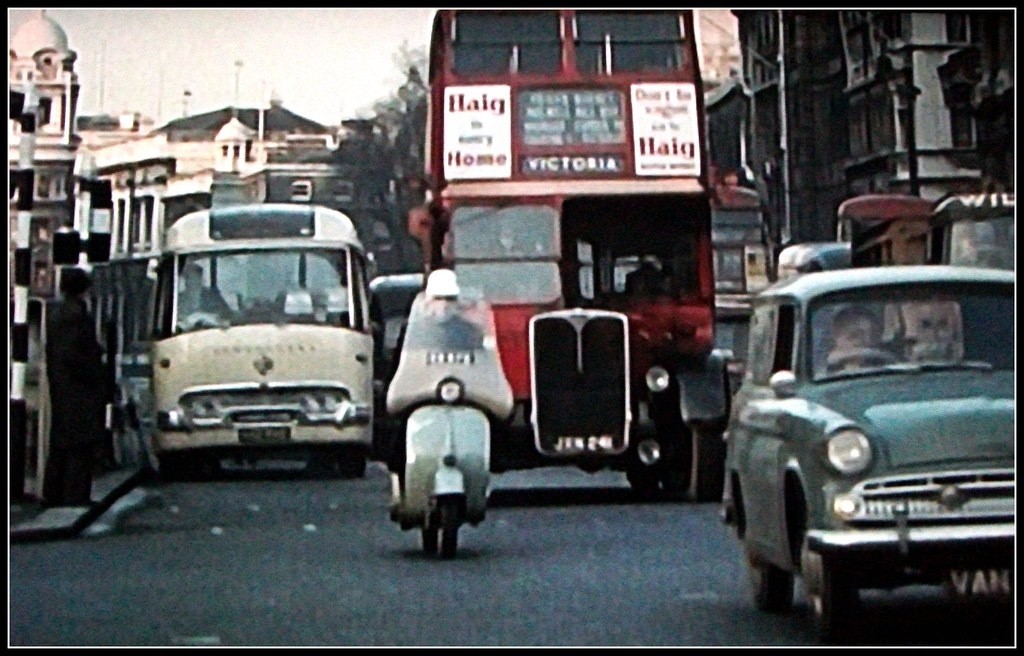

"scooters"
[384,289,513,561]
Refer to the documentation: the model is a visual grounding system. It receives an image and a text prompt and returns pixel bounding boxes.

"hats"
[60,269,91,296]
[425,271,459,302]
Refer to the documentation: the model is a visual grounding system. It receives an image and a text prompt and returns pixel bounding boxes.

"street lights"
[875,22,929,204]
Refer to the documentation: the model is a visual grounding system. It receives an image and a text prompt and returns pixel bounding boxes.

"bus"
[709,177,776,380]
[75,203,378,479]
[420,10,730,500]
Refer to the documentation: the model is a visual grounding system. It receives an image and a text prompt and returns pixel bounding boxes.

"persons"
[163,262,236,342]
[378,266,489,526]
[40,266,123,505]
[814,306,900,382]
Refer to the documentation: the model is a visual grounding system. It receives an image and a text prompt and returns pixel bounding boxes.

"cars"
[776,243,853,280]
[720,264,1013,636]
[365,274,428,355]
